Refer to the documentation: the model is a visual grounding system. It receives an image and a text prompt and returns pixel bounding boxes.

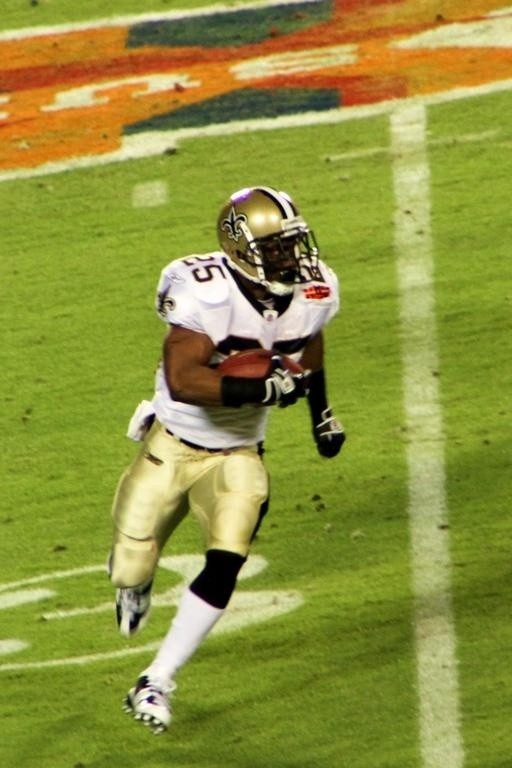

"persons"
[106,185,346,735]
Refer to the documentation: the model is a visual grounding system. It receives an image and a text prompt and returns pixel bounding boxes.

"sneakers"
[122,676,178,735]
[115,579,153,638]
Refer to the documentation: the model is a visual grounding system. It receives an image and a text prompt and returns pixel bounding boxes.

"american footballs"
[216,350,304,377]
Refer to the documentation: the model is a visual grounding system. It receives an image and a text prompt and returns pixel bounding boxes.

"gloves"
[220,350,312,408]
[307,369,345,457]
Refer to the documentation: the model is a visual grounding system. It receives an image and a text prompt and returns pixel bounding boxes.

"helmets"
[217,186,326,296]
